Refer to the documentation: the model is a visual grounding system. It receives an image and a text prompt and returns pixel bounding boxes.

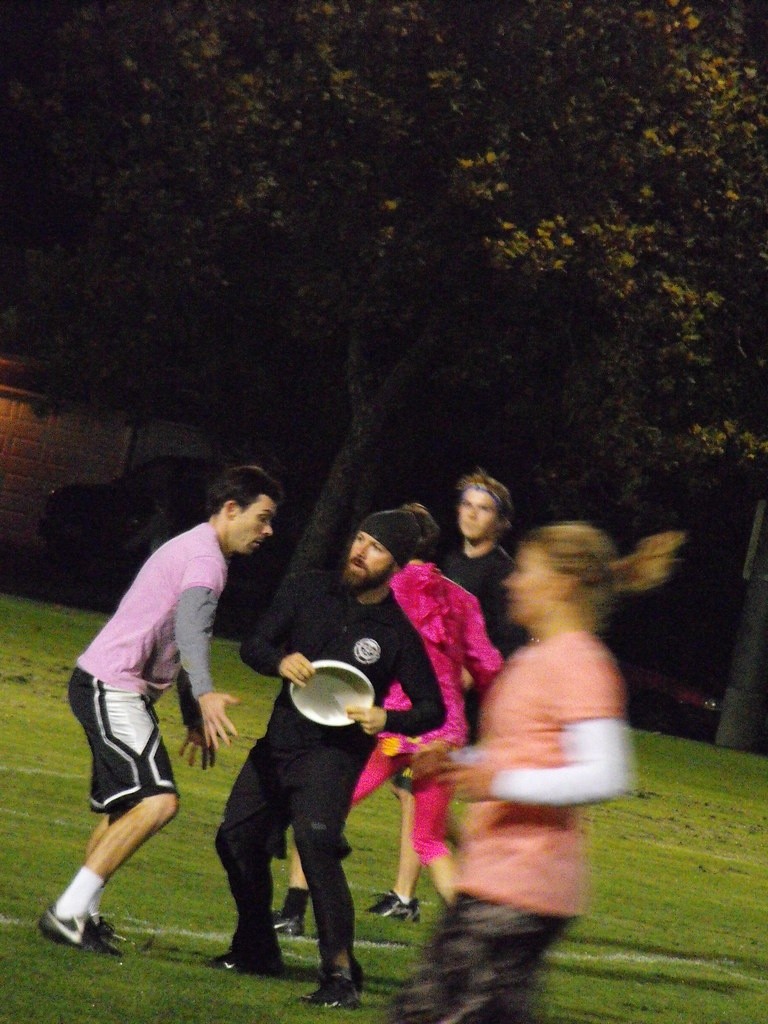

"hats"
[359,510,421,569]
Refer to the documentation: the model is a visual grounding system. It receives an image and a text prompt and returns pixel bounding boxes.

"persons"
[213,473,535,1007]
[37,463,278,958]
[387,521,688,1023]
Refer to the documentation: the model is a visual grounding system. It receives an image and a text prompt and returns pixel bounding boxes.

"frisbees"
[288,659,375,728]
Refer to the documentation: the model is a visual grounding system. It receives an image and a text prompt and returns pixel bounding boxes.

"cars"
[37,468,209,563]
[619,651,721,739]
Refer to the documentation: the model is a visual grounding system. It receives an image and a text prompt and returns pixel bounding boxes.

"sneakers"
[301,971,361,1011]
[38,903,123,958]
[213,946,286,978]
[366,890,420,923]
[95,916,127,944]
[271,910,304,936]
